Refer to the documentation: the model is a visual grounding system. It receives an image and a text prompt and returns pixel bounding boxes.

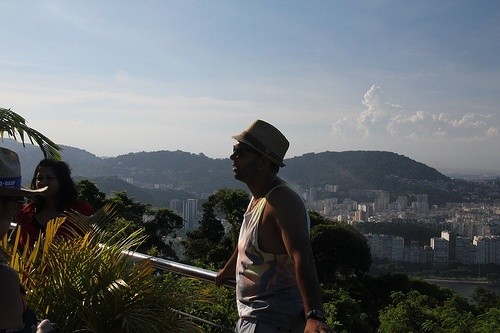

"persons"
[1,146,58,333]
[13,157,95,261]
[215,119,328,333]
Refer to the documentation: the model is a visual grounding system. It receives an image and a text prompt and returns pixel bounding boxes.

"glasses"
[232,143,264,161]
[34,174,55,180]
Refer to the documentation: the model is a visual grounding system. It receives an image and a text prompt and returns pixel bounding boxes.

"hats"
[0,146,48,197]
[230,118,289,167]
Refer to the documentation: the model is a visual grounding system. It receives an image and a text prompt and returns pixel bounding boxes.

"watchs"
[305,309,326,322]
